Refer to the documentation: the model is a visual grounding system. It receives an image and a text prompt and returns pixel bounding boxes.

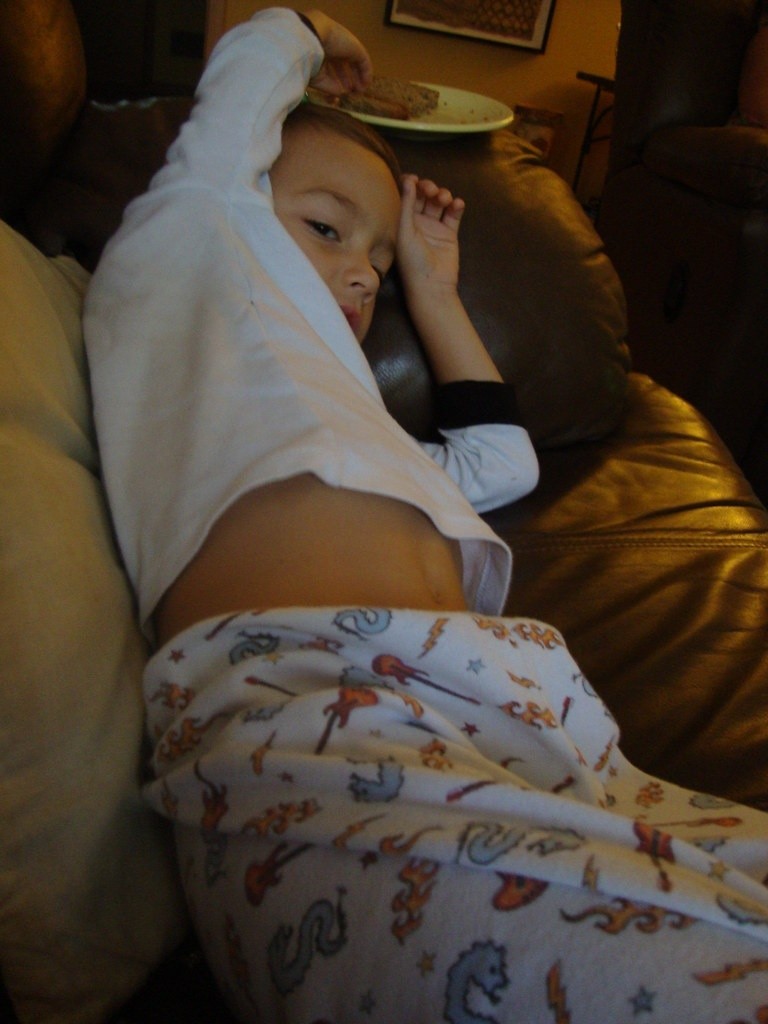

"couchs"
[0,0,767,1024]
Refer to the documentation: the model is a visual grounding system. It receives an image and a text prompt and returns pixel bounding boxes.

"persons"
[80,5,768,1024]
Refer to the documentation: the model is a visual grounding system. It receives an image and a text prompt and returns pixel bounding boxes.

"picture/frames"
[383,0,558,55]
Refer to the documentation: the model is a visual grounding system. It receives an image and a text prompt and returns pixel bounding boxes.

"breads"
[339,77,440,120]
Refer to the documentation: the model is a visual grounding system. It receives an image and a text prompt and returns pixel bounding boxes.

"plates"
[305,74,515,132]
